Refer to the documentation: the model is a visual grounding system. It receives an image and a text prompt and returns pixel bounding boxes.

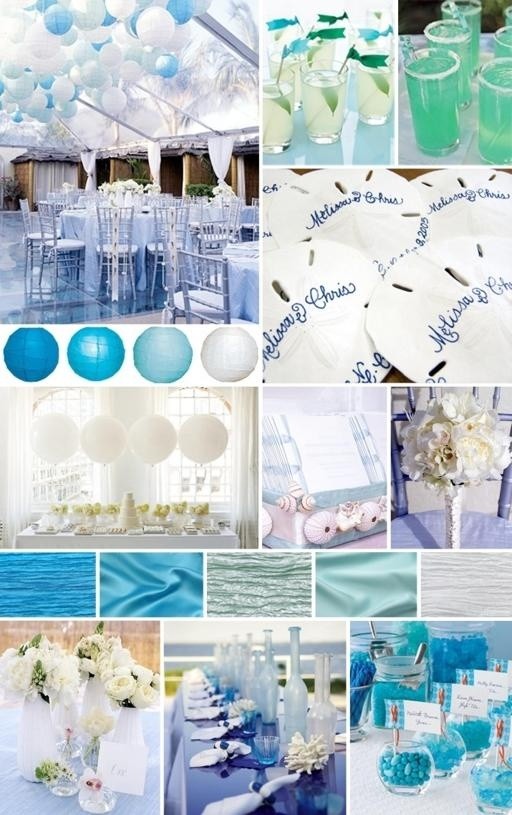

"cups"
[400,0,510,164]
[350,622,511,815]
[201,664,279,766]
[262,42,393,155]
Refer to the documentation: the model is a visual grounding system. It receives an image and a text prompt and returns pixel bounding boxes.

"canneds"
[350,622,494,730]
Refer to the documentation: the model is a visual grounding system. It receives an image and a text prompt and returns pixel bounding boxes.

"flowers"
[396,389,512,505]
[282,732,331,780]
[0,621,160,815]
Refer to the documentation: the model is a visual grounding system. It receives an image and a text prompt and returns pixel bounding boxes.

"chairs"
[15,175,258,323]
[395,419,512,519]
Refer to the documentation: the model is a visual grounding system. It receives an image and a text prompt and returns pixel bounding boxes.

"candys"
[378,750,431,786]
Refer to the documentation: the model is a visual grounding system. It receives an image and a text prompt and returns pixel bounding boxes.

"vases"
[295,771,329,814]
[439,484,464,549]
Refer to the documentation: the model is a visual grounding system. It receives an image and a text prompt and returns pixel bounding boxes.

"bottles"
[213,625,339,759]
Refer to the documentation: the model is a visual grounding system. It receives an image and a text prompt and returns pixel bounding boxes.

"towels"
[184,685,296,814]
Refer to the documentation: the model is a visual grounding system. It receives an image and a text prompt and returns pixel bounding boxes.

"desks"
[166,662,346,815]
[13,516,239,549]
[390,510,512,549]
